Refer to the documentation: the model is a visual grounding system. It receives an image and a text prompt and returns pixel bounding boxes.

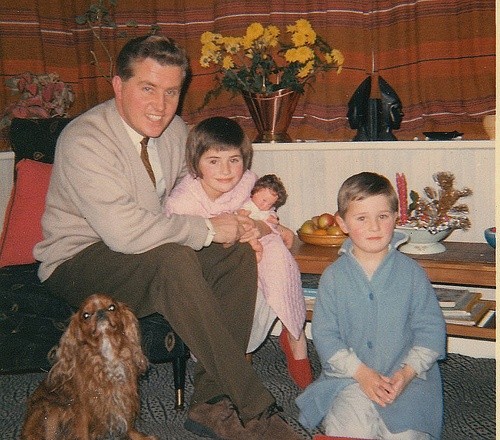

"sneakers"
[183,398,252,440]
[245,407,303,440]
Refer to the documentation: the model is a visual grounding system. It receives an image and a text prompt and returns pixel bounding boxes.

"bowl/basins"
[423,131,465,141]
[296,228,348,247]
[484,227,496,248]
[483,114,495,140]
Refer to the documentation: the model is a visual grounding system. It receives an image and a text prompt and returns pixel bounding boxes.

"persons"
[238,175,294,263]
[294,172,446,440]
[33,34,304,440]
[164,116,312,389]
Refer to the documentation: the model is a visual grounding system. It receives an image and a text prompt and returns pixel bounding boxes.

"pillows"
[0,158,55,268]
[0,151,16,257]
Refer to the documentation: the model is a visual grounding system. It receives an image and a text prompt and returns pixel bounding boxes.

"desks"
[282,232,496,342]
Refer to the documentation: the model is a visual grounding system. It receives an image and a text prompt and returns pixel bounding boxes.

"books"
[301,273,322,310]
[432,288,496,327]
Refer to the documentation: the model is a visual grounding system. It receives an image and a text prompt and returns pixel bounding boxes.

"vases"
[233,81,307,144]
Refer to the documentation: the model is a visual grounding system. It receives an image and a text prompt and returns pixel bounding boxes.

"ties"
[139,137,156,191]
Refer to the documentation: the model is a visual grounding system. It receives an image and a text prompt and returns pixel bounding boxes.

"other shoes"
[280,329,312,391]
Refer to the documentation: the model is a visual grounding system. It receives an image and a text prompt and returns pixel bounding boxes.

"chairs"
[0,114,194,410]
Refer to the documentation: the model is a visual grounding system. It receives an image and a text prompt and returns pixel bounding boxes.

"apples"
[300,214,343,236]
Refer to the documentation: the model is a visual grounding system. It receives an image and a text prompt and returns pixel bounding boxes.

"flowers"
[193,18,343,115]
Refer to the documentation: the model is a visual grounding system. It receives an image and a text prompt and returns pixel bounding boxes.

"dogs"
[21,294,158,440]
